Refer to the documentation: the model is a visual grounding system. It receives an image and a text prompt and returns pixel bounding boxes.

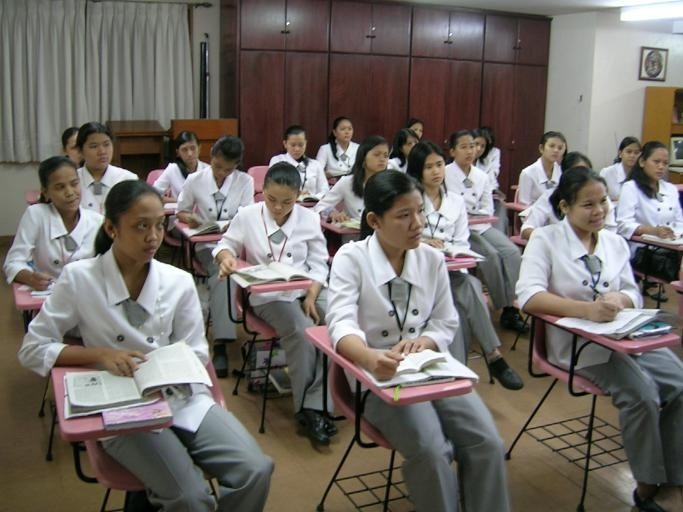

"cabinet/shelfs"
[480,10,551,195]
[327,0,411,146]
[220,0,331,170]
[411,2,485,158]
[643,86,683,181]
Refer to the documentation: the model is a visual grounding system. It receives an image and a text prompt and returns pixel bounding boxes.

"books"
[553,305,676,339]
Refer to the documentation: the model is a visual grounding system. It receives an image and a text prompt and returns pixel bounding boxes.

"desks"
[107,120,168,174]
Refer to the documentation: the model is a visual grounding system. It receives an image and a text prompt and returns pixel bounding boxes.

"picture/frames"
[639,47,668,81]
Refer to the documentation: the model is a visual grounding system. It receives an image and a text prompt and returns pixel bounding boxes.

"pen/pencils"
[589,285,603,300]
[28,262,56,283]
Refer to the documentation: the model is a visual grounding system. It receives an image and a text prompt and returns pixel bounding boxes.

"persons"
[18,179,274,512]
[515,165,683,510]
[325,170,510,511]
[3,116,532,447]
[519,132,683,301]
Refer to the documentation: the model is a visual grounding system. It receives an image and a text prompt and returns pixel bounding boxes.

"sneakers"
[298,410,337,446]
[488,357,524,390]
[630,488,666,511]
[212,348,229,377]
[499,310,530,332]
[652,292,668,302]
